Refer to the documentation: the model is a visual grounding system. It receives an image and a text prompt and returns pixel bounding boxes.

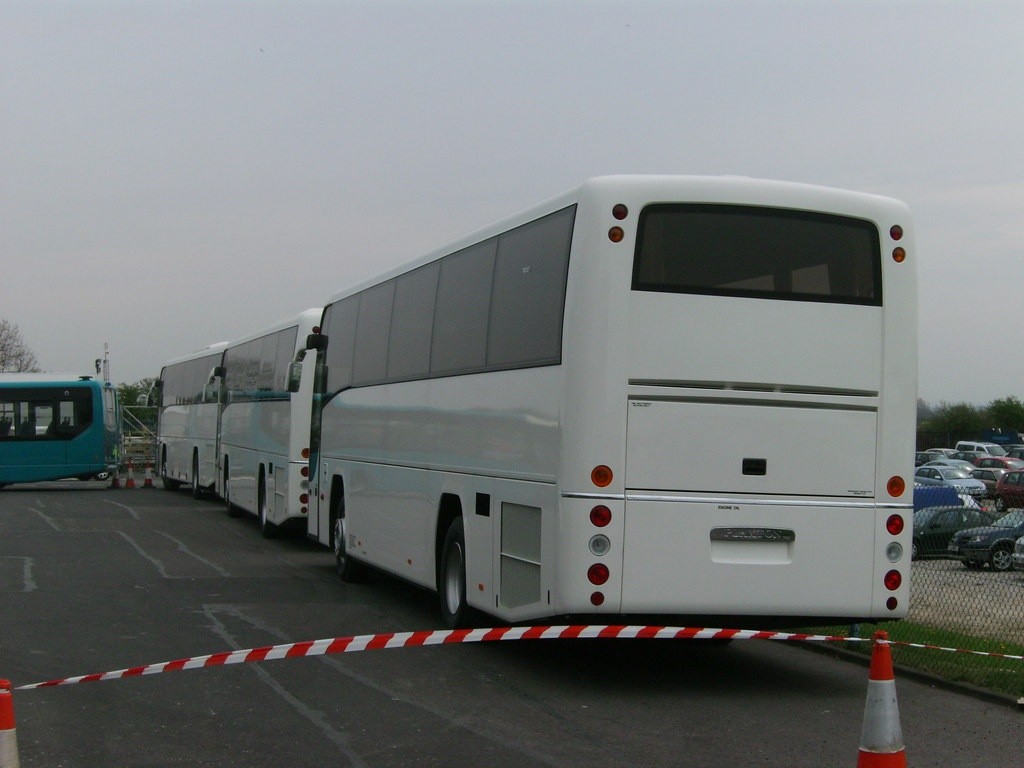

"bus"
[0,373,122,490]
[144,307,326,538]
[284,173,918,646]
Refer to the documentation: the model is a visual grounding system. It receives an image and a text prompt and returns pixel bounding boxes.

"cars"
[912,440,1024,571]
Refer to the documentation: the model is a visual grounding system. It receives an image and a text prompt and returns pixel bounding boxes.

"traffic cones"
[856,629,908,768]
[125,457,135,488]
[141,458,155,488]
[107,470,121,489]
[0,679,19,768]
